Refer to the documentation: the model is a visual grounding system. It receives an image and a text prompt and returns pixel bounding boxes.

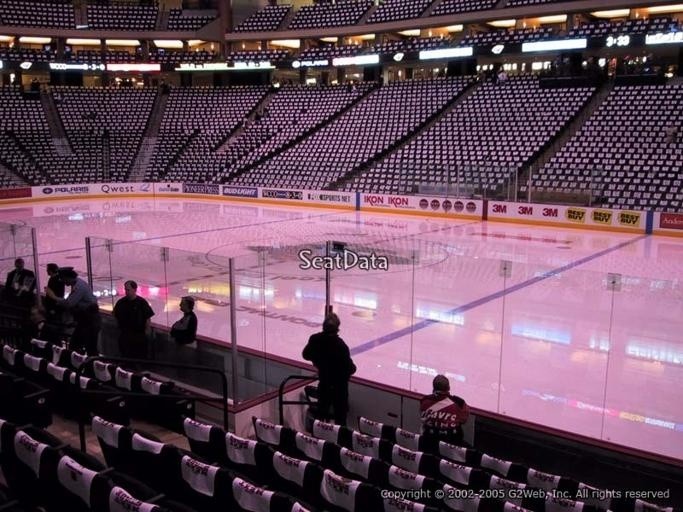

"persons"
[0,257,100,356]
[168,296,198,348]
[303,314,356,425]
[113,279,154,359]
[419,375,471,454]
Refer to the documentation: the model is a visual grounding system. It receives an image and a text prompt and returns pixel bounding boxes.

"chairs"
[0,334,664,511]
[0,0,682,224]
